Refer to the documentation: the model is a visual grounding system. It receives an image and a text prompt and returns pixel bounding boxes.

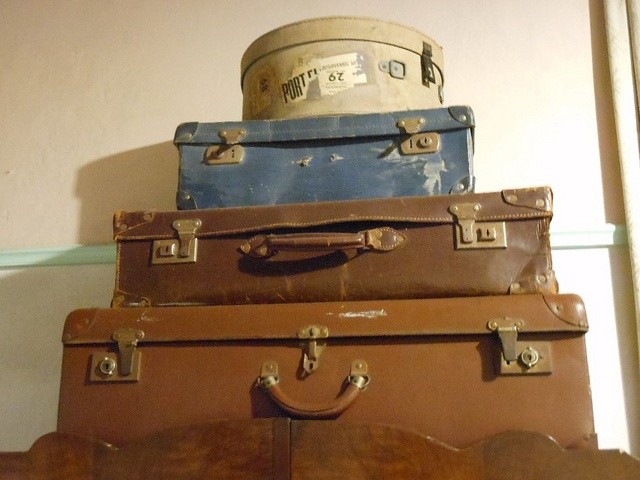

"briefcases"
[173,106,477,210]
[110,187,561,296]
[55,295,597,451]
